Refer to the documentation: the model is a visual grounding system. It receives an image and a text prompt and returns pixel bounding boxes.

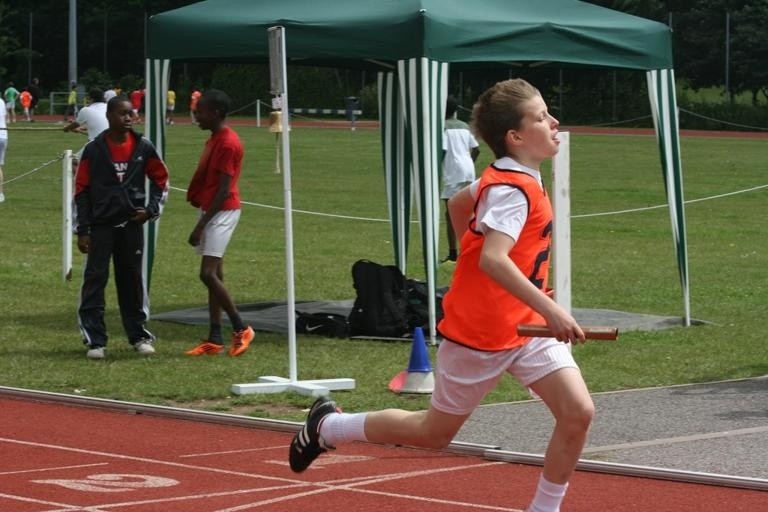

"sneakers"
[135,341,156,354]
[289,395,344,473]
[85,346,108,359]
[228,325,256,356]
[439,256,458,264]
[0,193,5,203]
[183,341,226,356]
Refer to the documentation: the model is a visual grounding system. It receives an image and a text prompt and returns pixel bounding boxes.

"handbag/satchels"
[294,309,349,340]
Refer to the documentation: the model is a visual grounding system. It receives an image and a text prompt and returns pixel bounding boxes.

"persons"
[288,77,594,512]
[20,86,33,122]
[0,96,9,202]
[64,81,145,122]
[189,88,202,125]
[64,88,110,159]
[73,94,170,359]
[4,82,20,123]
[166,86,176,125]
[26,78,40,122]
[438,94,480,265]
[182,89,255,357]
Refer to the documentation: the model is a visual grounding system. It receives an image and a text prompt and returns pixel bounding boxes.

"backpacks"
[347,258,450,338]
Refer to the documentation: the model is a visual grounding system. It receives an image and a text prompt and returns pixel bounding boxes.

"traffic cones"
[396,326,437,398]
